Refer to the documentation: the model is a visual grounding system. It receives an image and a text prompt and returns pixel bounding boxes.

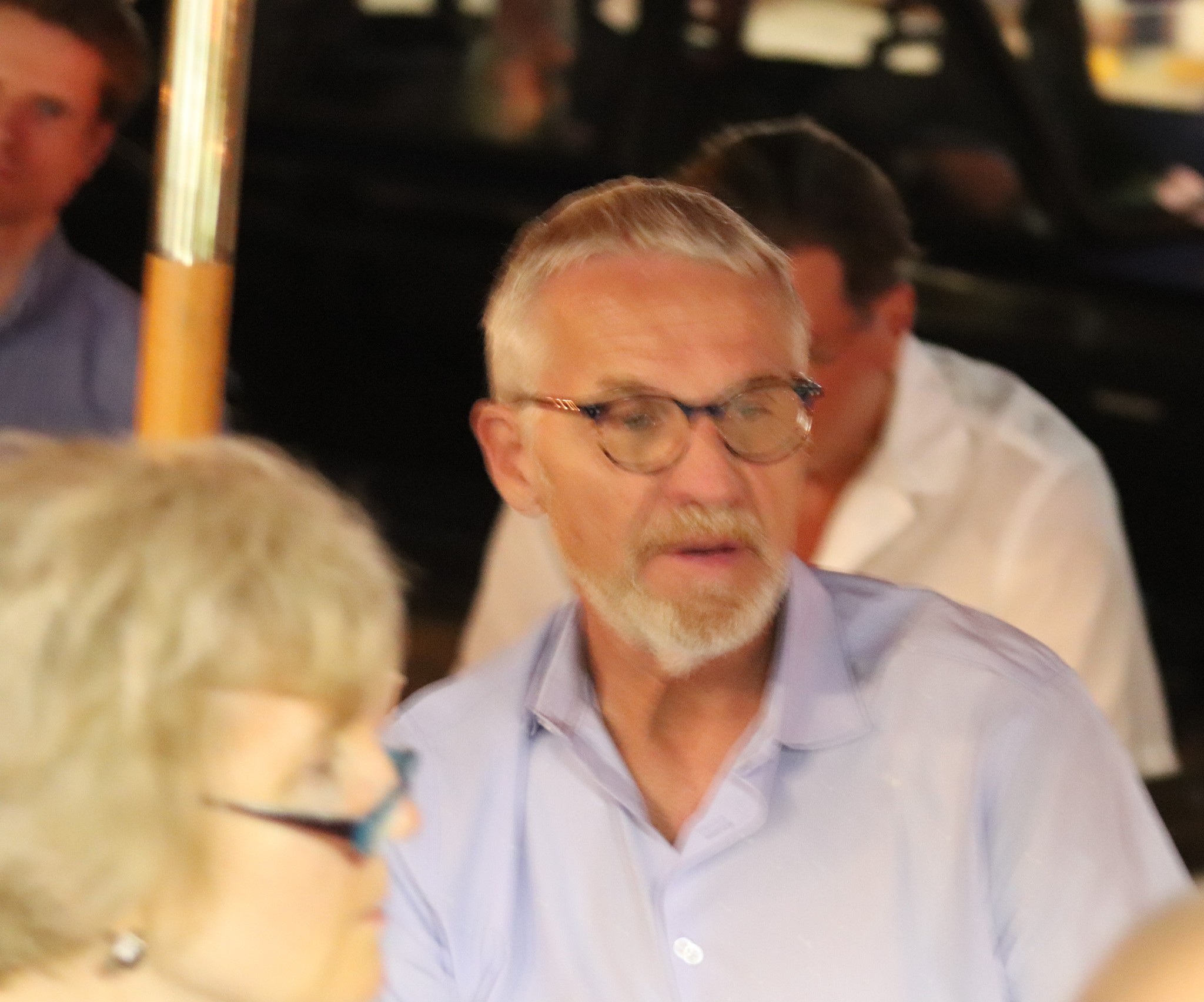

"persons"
[0,0,245,459]
[0,416,421,1001]
[312,165,1203,1002]
[437,112,1183,821]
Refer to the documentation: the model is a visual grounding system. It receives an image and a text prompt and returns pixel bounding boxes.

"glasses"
[512,366,825,477]
[200,742,427,860]
